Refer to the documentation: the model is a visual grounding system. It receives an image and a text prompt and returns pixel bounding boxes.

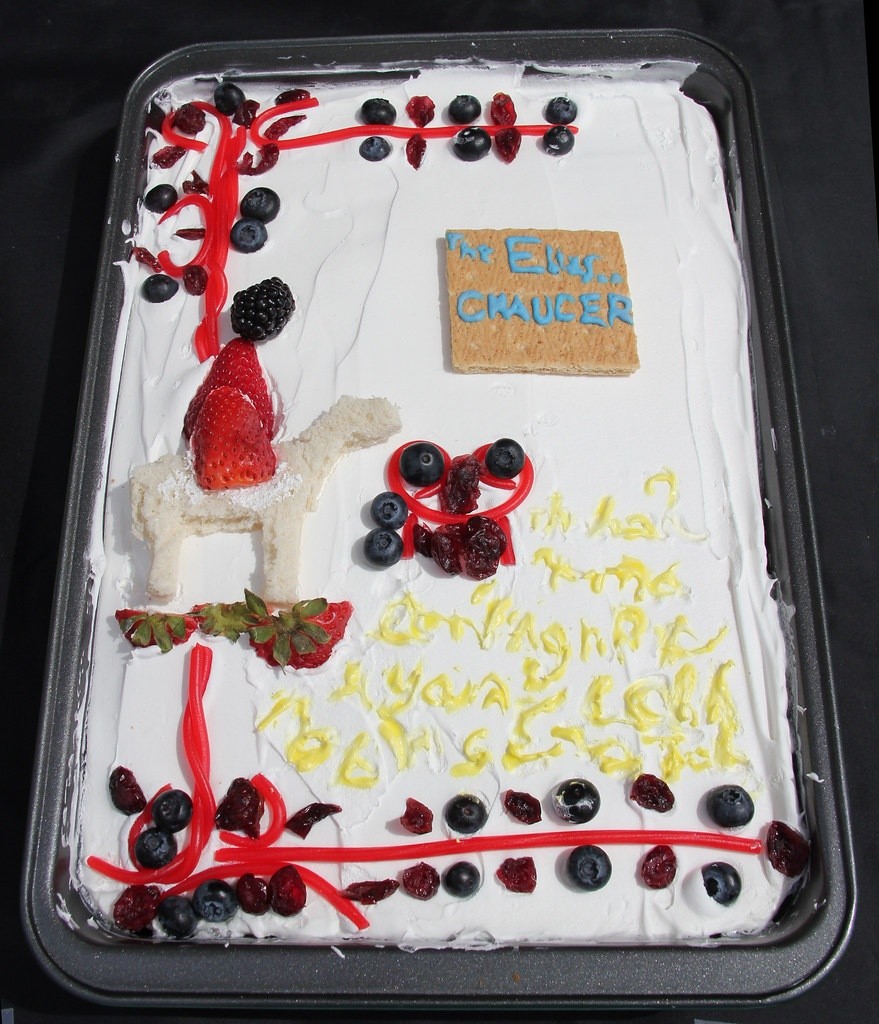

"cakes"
[65,65,811,946]
